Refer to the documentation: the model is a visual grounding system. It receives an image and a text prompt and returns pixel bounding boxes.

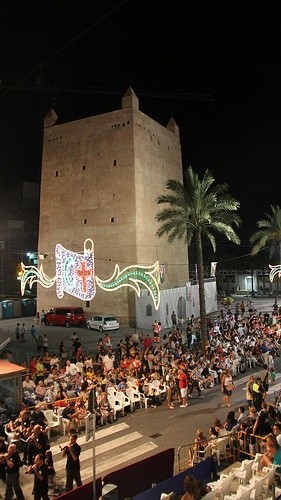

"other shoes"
[179,405,186,408]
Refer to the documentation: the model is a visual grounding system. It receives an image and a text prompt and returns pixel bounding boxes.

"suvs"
[44,306,85,328]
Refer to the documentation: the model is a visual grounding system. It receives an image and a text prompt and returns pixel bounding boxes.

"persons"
[1,285,280,500]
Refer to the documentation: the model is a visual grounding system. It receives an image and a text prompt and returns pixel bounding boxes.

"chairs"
[190,427,281,500]
[24,353,257,441]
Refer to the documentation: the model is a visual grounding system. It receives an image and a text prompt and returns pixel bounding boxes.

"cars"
[87,315,120,333]
